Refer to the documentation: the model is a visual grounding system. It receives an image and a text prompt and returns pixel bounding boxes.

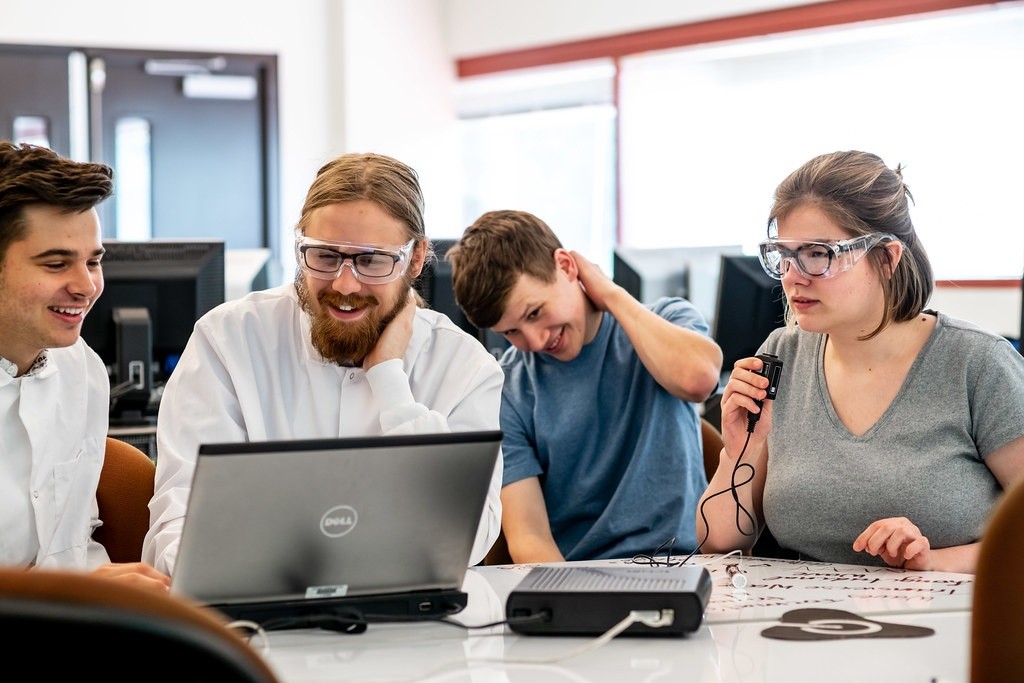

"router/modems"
[506,563,712,637]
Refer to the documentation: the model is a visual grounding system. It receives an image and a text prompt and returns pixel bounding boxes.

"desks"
[246,555,977,683]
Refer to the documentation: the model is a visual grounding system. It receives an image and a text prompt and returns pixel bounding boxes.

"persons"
[443,209,722,563]
[697,151,1024,578]
[139,152,504,577]
[0,138,172,590]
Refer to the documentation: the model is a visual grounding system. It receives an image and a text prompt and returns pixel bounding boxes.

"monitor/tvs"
[713,254,792,372]
[81,238,225,422]
[614,249,691,305]
[416,239,506,365]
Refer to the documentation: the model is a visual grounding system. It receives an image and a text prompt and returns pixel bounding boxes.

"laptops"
[157,428,504,634]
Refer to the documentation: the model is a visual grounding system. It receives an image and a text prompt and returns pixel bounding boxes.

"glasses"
[295,227,415,285]
[757,232,905,281]
